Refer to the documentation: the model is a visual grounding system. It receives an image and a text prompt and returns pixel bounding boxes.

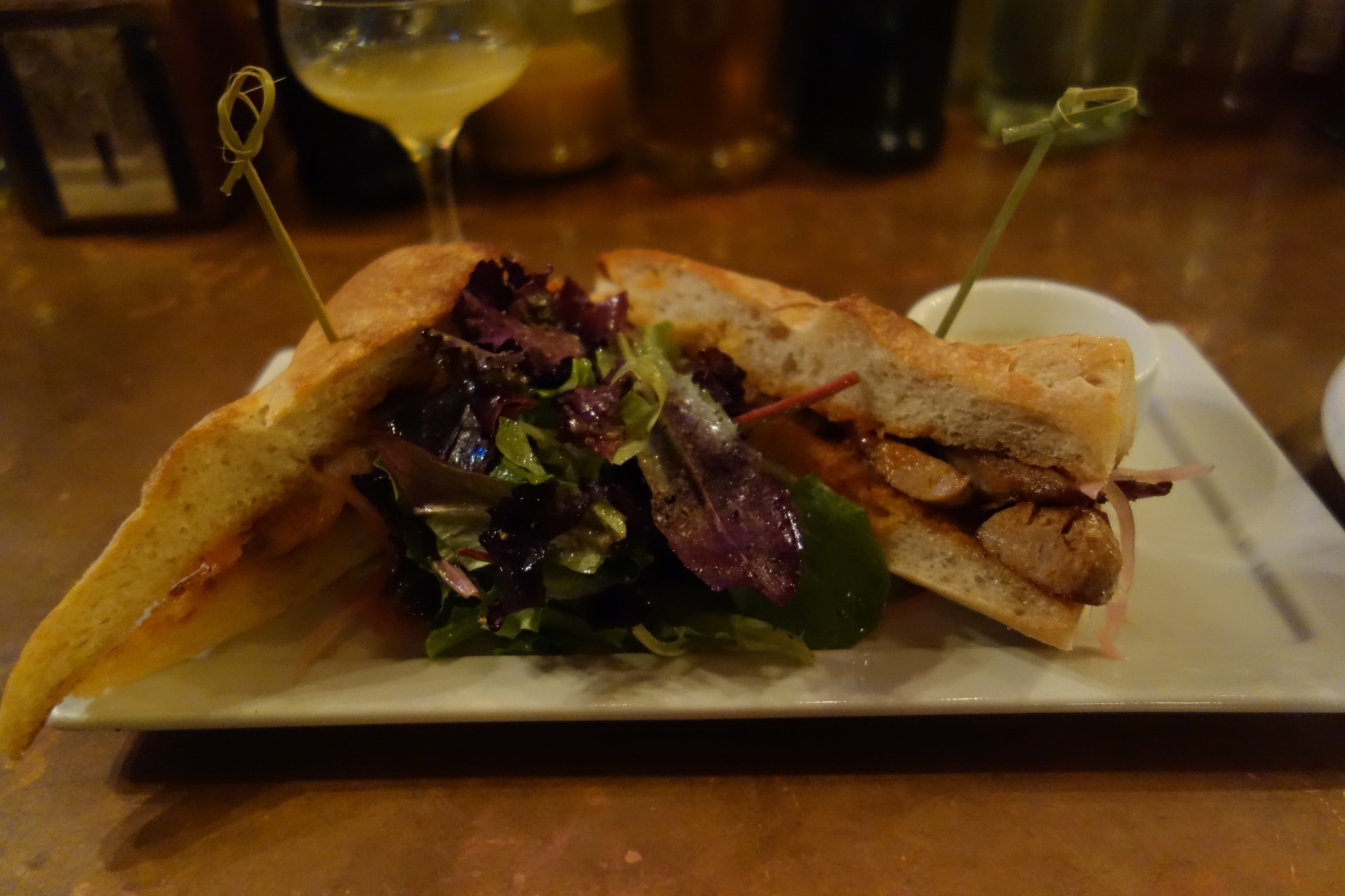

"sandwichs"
[0,243,492,765]
[597,249,1217,654]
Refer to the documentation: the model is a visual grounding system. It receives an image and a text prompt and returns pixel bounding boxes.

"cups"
[1322,357,1345,477]
[471,2,636,176]
[635,4,781,185]
[255,2,421,210]
[782,0,959,173]
[904,277,1162,380]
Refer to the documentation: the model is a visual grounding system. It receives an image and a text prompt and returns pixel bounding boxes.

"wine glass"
[276,0,532,247]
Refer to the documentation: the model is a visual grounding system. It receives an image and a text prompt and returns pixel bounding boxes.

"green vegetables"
[350,252,889,668]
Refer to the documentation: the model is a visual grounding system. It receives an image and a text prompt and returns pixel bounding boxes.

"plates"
[44,323,1345,732]
